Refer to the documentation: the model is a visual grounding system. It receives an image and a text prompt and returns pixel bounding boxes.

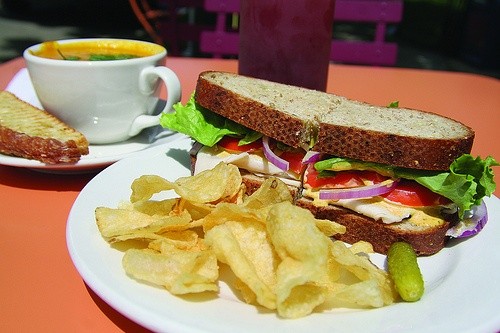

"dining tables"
[0,58,500,333]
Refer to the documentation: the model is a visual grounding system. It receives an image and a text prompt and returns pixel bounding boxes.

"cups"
[237,0,337,92]
[24,39,181,146]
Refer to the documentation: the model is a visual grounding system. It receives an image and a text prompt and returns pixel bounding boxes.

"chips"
[95,162,396,318]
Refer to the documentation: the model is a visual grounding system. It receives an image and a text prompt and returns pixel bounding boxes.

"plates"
[66,130,500,332]
[0,66,177,172]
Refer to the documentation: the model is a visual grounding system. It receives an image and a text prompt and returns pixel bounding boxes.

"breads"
[0,89,90,165]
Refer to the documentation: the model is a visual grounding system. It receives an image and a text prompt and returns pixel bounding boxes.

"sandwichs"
[160,70,500,255]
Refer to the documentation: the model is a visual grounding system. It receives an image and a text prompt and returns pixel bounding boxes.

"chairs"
[199,0,403,67]
